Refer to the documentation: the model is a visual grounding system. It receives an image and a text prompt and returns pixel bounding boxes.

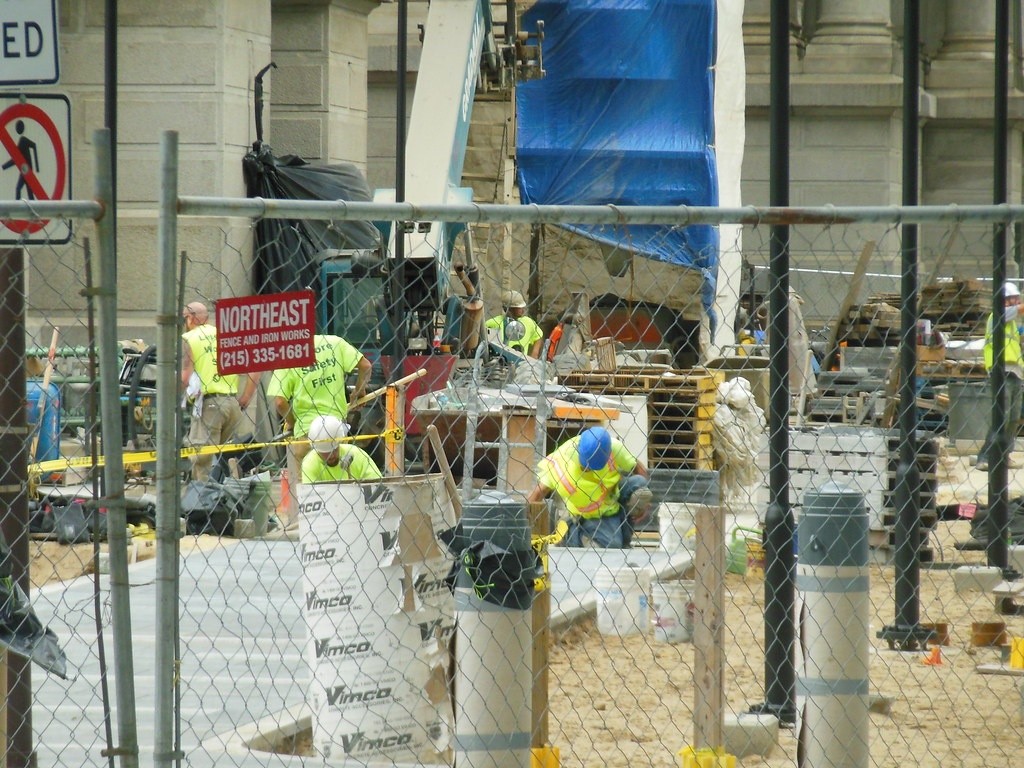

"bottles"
[433,333,441,355]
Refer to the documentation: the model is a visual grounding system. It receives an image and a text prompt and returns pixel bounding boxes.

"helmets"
[308,415,345,453]
[579,427,612,471]
[183,302,209,325]
[502,290,526,308]
[1004,283,1021,296]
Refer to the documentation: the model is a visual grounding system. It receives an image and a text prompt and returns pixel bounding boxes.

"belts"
[204,392,239,399]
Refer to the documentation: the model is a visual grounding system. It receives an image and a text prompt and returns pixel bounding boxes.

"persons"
[527,426,650,548]
[267,335,371,483]
[301,416,383,484]
[180,302,262,481]
[485,290,543,359]
[975,282,1024,471]
[736,306,757,357]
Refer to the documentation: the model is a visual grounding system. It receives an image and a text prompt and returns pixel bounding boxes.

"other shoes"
[976,462,988,470]
[1008,459,1022,469]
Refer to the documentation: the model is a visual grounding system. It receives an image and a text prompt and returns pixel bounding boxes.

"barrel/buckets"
[656,502,709,563]
[221,477,269,536]
[595,567,650,637]
[648,579,701,644]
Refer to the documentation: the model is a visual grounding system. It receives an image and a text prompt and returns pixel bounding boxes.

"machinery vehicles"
[320,0,771,470]
